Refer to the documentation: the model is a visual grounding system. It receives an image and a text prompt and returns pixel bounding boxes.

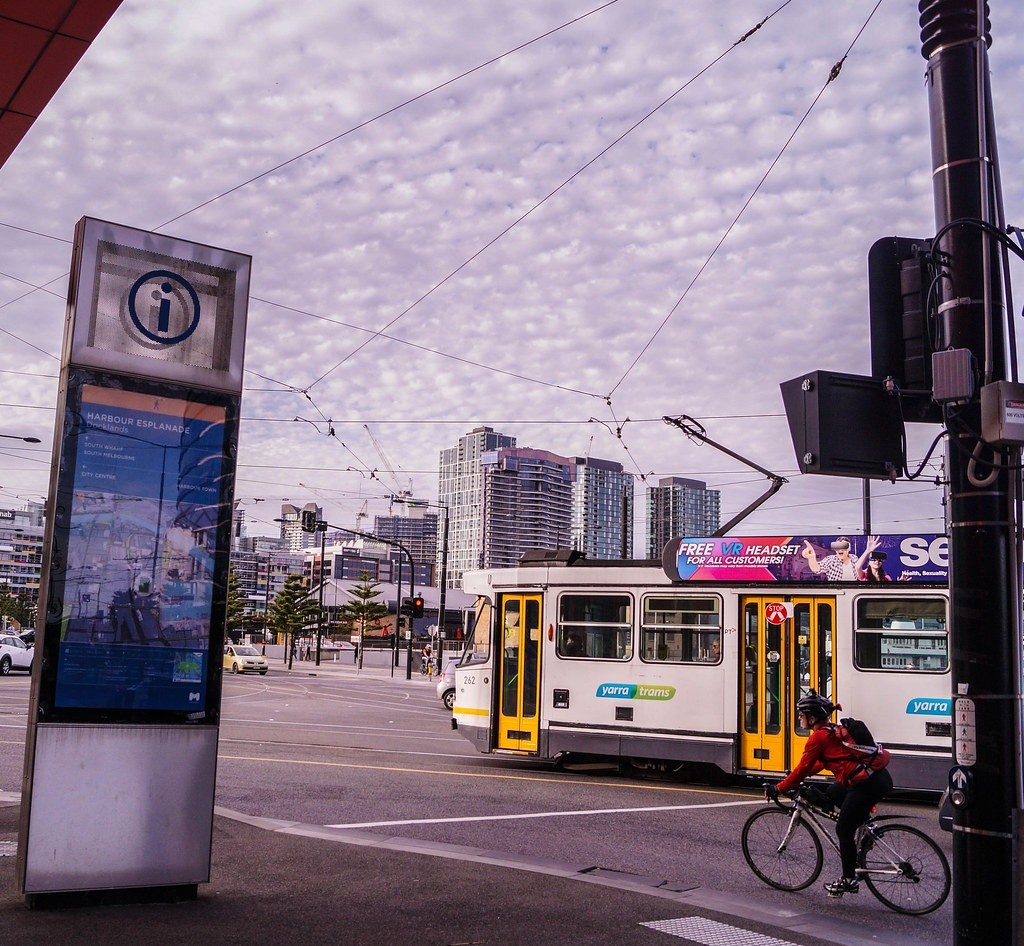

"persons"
[293,644,298,662]
[762,693,894,896]
[712,638,720,662]
[565,634,582,650]
[801,537,862,581]
[304,645,312,662]
[855,536,912,581]
[421,644,435,669]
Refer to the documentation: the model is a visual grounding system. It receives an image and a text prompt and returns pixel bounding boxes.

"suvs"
[0,634,36,675]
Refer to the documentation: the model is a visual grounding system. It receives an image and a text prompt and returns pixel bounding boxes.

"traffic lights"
[413,597,425,619]
[82,595,91,603]
[400,597,413,619]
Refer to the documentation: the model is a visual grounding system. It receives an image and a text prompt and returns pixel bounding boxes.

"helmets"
[796,695,833,714]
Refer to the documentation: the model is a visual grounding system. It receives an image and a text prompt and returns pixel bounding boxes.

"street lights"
[271,518,415,680]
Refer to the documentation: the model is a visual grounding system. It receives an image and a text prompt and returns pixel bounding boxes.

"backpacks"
[817,718,892,771]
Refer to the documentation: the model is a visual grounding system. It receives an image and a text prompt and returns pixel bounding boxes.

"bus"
[451,533,996,799]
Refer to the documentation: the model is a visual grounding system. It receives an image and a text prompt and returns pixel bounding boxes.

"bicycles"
[420,657,439,677]
[742,770,952,916]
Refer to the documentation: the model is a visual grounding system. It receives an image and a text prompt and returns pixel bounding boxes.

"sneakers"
[860,823,884,839]
[823,877,859,893]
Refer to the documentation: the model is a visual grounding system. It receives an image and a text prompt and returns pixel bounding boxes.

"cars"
[20,630,35,643]
[224,645,268,675]
[437,660,462,710]
[319,641,356,649]
[0,630,19,638]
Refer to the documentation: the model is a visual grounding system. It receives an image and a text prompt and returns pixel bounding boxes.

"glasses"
[713,645,719,648]
